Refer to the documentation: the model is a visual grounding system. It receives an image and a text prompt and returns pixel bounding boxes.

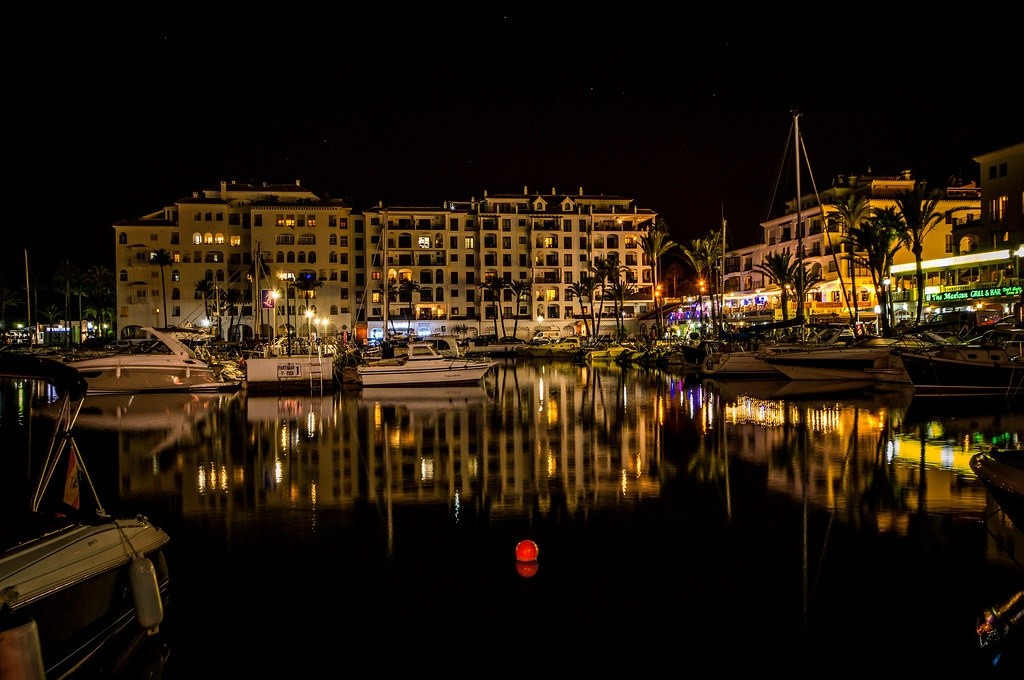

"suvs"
[471,333,497,346]
[555,337,580,348]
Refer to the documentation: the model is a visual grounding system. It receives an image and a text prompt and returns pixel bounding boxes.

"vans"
[533,331,561,342]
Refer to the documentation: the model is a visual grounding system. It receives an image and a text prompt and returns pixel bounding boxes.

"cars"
[499,336,526,344]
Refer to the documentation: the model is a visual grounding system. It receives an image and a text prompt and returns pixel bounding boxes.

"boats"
[40,393,239,456]
[970,448,1024,535]
[516,108,1024,387]
[703,374,917,404]
[0,352,176,680]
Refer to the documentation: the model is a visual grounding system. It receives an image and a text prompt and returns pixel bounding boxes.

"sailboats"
[0,205,501,395]
[246,395,349,510]
[358,387,495,559]
